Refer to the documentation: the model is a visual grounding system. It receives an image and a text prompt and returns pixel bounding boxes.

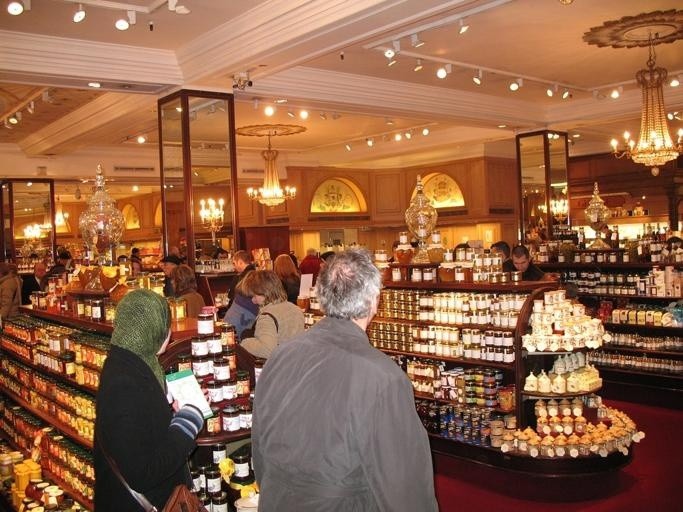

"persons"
[223,249,329,360]
[162,255,180,296]
[0,259,24,330]
[252,246,439,511]
[92,287,204,512]
[131,247,140,273]
[491,241,512,270]
[26,265,45,304]
[50,253,75,273]
[172,263,206,321]
[508,245,543,278]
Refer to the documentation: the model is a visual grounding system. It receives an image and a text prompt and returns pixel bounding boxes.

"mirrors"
[518,131,571,250]
[1,179,55,274]
[160,89,239,275]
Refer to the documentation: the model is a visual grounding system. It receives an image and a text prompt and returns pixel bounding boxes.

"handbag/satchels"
[161,484,208,512]
[238,329,267,368]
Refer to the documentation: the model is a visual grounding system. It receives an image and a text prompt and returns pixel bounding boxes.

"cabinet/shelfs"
[297,279,637,505]
[2,303,261,510]
[533,247,683,412]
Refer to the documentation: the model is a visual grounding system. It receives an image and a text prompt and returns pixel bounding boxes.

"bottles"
[489,382,637,458]
[604,331,682,351]
[525,352,586,392]
[576,226,586,250]
[612,223,619,250]
[585,350,683,375]
[634,223,660,262]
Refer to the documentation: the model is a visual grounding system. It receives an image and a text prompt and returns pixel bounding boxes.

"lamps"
[254,97,432,150]
[610,28,682,177]
[385,20,627,102]
[200,196,224,248]
[246,134,297,212]
[540,200,567,229]
[8,0,190,33]
[6,91,53,129]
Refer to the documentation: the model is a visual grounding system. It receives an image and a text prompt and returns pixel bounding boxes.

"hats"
[160,255,181,265]
